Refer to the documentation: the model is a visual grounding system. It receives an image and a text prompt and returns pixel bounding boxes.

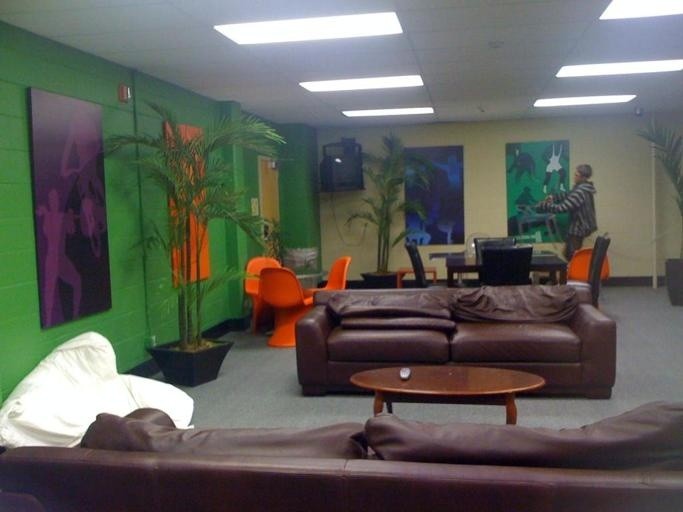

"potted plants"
[340,131,433,289]
[101,94,289,387]
[632,117,682,307]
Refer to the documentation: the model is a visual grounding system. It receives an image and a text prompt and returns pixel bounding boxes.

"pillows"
[0,332,194,450]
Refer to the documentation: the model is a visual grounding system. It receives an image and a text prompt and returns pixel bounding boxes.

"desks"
[348,364,546,426]
[443,255,567,286]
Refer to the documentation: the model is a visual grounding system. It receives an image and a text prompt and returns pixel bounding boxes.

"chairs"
[244,253,352,348]
[474,236,517,263]
[404,237,465,288]
[567,249,610,283]
[479,245,535,286]
[540,234,610,309]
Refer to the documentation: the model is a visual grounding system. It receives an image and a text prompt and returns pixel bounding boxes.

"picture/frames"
[26,85,113,332]
[400,143,465,246]
[504,139,574,247]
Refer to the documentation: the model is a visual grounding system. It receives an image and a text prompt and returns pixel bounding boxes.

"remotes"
[399,367,411,381]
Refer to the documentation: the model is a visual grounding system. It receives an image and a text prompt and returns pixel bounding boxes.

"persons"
[537,164,598,259]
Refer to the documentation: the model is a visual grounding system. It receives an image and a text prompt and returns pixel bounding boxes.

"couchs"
[1,402,681,512]
[293,281,618,400]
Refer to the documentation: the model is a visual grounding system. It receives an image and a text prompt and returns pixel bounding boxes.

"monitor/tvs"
[319,152,364,192]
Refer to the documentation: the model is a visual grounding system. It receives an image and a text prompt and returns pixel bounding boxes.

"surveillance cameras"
[635,107,643,116]
